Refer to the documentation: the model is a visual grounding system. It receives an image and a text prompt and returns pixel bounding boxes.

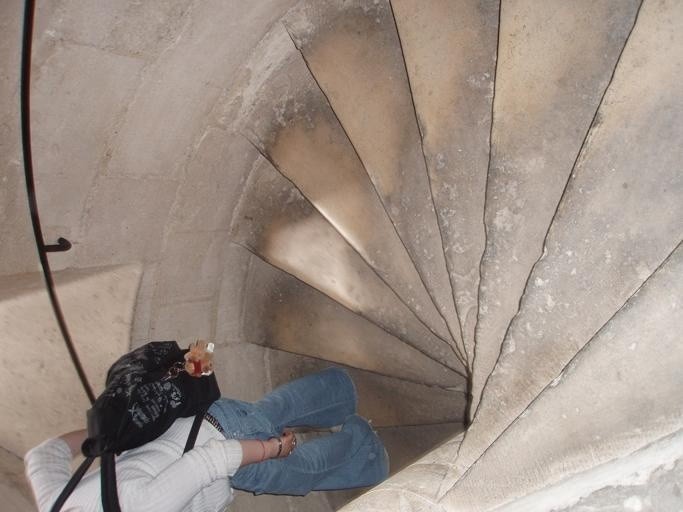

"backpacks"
[49,341,221,512]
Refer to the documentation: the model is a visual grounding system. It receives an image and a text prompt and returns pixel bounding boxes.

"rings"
[292,438,296,445]
[288,449,292,455]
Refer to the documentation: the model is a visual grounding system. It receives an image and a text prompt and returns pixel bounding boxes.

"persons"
[25,366,391,512]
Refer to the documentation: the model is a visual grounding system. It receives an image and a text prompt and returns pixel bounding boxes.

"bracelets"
[267,436,282,459]
[257,439,266,462]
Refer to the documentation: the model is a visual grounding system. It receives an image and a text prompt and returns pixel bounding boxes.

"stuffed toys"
[184,340,214,378]
